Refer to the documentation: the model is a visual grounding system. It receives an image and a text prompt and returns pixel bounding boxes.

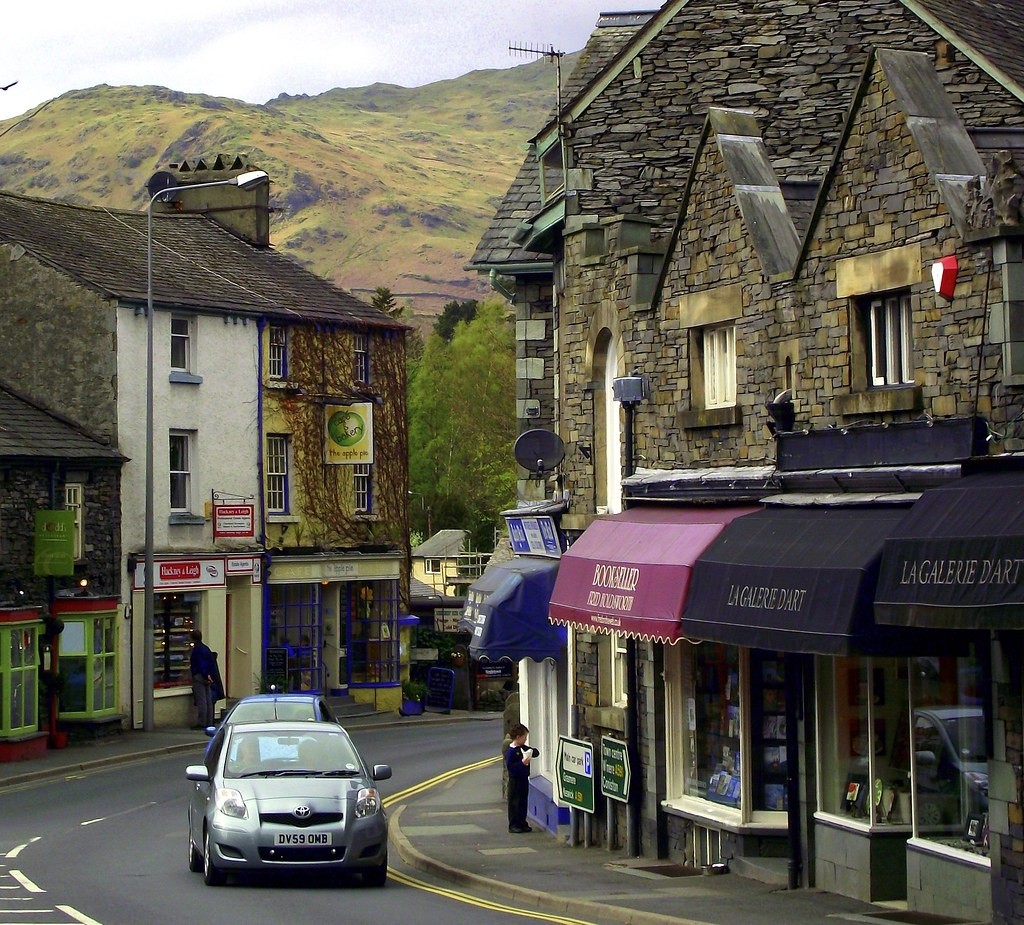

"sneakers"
[509,823,532,833]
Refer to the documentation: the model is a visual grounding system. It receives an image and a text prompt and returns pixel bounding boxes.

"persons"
[280,635,312,659]
[190,630,215,730]
[228,737,261,772]
[505,723,539,833]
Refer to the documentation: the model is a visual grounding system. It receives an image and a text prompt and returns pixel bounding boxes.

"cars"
[186,716,392,888]
[204,692,336,756]
[913,703,987,809]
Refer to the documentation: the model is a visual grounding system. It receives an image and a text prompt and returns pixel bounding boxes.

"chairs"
[296,739,322,767]
[235,742,261,764]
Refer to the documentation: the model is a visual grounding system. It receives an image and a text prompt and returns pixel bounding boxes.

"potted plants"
[402,679,426,716]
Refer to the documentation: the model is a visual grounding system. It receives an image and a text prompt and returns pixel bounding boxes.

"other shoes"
[190,724,215,730]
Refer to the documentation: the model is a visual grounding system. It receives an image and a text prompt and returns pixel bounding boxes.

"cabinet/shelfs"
[154,607,192,672]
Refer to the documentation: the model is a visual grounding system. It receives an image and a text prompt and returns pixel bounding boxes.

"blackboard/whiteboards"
[427,667,454,706]
[264,648,288,682]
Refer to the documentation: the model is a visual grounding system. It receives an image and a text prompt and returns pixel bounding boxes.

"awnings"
[680,509,910,657]
[469,561,561,663]
[873,473,1024,630]
[457,558,550,634]
[548,507,764,645]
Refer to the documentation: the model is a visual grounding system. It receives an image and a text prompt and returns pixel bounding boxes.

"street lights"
[142,171,269,731]
[429,595,444,632]
